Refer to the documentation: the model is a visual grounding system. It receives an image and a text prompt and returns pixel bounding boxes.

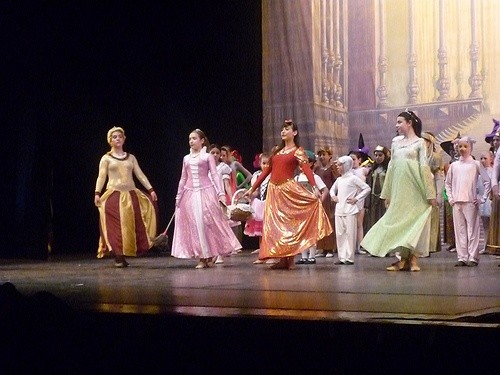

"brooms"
[149,212,175,253]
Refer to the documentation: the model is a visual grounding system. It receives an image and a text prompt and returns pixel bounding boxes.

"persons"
[359,110,436,271]
[242,119,334,270]
[205,119,500,266]
[94,126,158,268]
[171,129,242,269]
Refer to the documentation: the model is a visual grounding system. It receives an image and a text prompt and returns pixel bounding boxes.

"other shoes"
[115,261,128,267]
[271,262,287,268]
[215,258,224,264]
[467,261,478,267]
[334,261,344,265]
[315,253,325,257]
[195,262,210,268]
[344,261,354,265]
[252,258,277,264]
[304,258,317,264]
[295,258,308,264]
[454,261,466,266]
[286,264,296,270]
[326,253,334,258]
[386,261,408,270]
[410,264,420,271]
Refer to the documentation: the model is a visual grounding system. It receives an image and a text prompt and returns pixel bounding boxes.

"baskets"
[229,190,251,222]
